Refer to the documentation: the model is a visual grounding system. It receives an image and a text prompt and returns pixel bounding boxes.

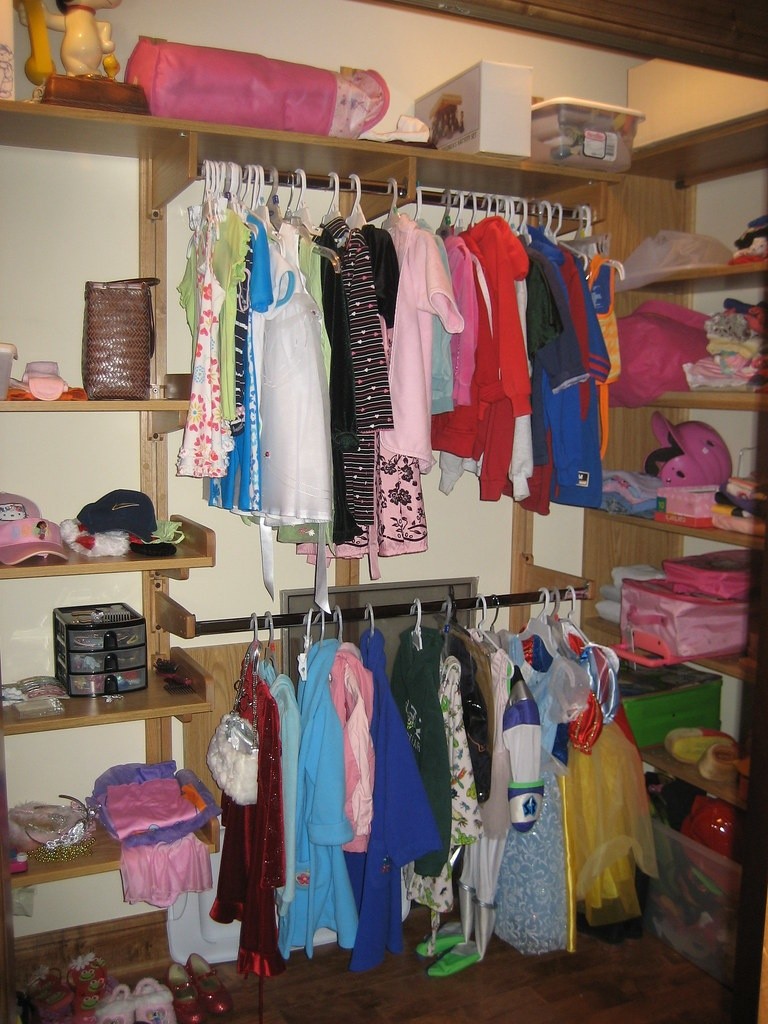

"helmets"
[643,411,734,490]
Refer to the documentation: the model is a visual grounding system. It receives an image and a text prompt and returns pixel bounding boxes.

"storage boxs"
[528,96,645,174]
[412,58,533,155]
[641,816,743,987]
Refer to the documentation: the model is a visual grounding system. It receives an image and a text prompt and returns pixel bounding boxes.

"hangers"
[242,583,592,685]
[199,158,626,283]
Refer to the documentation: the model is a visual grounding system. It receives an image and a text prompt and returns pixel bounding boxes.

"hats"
[0,519,68,567]
[78,488,159,543]
[0,492,40,521]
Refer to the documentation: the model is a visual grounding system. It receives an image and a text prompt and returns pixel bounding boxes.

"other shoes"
[665,728,738,764]
[95,983,133,1024]
[185,952,232,1015]
[24,951,108,1024]
[697,745,741,782]
[166,961,205,1024]
[135,977,176,1024]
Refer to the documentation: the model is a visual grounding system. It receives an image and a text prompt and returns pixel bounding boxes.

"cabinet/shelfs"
[575,101,767,807]
[0,94,222,998]
[144,106,627,881]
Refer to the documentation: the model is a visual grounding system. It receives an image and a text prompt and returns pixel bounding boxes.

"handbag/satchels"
[207,640,263,805]
